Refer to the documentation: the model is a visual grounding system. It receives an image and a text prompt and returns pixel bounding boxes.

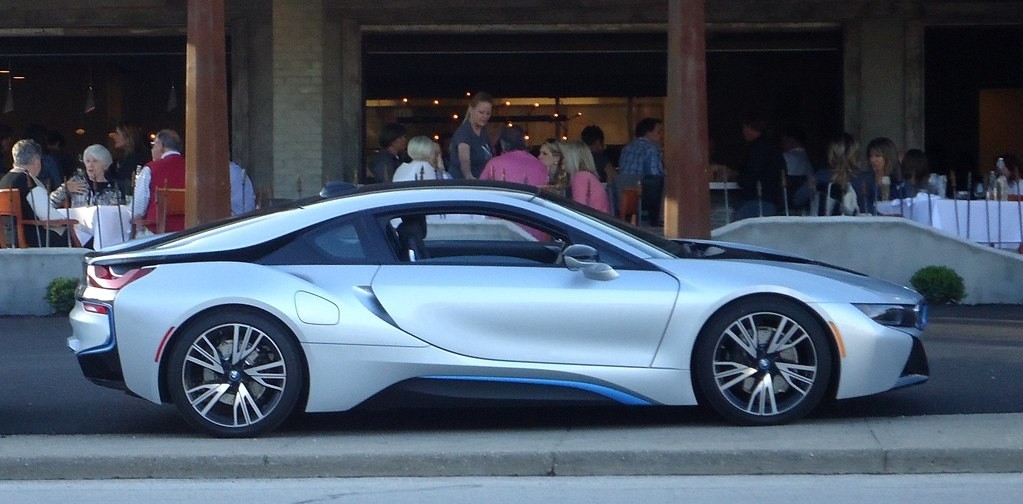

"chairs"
[614,173,665,236]
[0,188,82,248]
[401,214,427,262]
[129,185,186,239]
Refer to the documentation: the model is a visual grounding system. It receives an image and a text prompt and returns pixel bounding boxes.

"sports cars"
[64,175,931,441]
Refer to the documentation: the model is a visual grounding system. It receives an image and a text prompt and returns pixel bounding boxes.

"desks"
[709,181,744,189]
[56,205,130,251]
[874,197,1023,256]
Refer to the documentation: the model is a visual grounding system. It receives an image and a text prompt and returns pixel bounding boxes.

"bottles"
[996,158,1005,176]
[988,171,998,200]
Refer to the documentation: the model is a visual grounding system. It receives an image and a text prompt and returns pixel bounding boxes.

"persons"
[449,92,492,180]
[900,149,940,194]
[392,136,453,220]
[229,161,257,216]
[1009,167,1023,194]
[619,117,664,187]
[733,112,783,200]
[126,129,185,234]
[538,138,569,185]
[781,123,820,170]
[794,134,875,214]
[111,122,147,194]
[579,125,620,183]
[0,139,72,248]
[369,123,407,183]
[562,141,611,216]
[48,144,126,207]
[856,137,909,201]
[478,125,549,241]
[0,121,12,175]
